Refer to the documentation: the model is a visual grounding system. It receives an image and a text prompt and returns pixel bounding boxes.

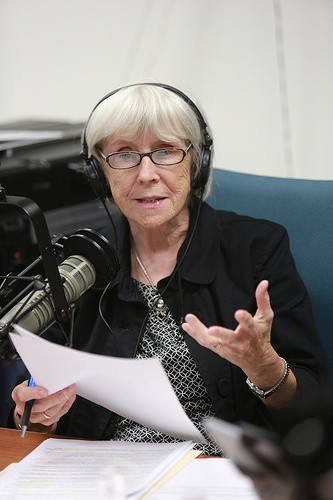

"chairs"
[206,169,333,365]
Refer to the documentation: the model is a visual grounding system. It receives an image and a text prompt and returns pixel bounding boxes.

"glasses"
[97,143,193,170]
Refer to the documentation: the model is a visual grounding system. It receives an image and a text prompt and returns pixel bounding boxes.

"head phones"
[80,82,213,202]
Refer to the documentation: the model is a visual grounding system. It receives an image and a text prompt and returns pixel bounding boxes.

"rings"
[215,344,222,350]
[42,412,52,419]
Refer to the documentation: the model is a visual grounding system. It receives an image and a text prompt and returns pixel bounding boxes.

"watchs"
[245,358,289,401]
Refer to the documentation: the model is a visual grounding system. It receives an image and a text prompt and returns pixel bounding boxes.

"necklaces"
[130,237,165,310]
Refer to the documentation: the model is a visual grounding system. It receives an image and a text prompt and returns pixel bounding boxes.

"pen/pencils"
[21,376,36,439]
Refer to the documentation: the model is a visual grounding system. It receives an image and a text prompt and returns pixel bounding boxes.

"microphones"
[0,225,120,356]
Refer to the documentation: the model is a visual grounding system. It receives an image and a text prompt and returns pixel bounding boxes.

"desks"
[0,426,333,500]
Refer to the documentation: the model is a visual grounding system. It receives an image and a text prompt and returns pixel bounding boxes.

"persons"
[0,84,333,453]
